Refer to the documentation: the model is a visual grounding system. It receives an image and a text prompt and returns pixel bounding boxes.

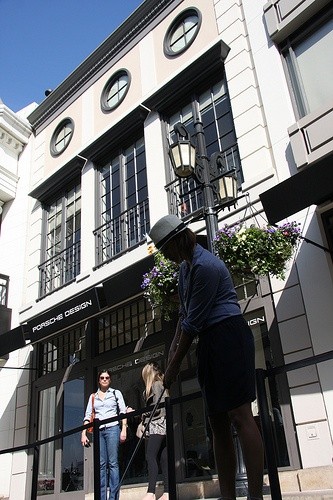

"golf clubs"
[111,386,167,493]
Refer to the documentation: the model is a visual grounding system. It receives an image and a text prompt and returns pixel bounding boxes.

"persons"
[148,215,263,500]
[136,362,169,500]
[81,370,126,500]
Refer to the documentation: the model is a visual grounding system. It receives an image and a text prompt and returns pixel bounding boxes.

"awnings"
[259,151,333,256]
[0,235,210,369]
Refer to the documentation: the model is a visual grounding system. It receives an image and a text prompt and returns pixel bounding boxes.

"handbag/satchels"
[87,393,95,433]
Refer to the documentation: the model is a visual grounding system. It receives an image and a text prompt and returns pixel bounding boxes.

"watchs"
[122,423,128,427]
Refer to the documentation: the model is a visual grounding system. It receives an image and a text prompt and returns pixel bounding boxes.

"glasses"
[100,376,110,380]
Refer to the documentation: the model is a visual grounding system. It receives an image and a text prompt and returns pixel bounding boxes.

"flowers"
[211,221,303,282]
[138,251,183,323]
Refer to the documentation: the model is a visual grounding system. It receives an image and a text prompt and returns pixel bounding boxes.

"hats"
[149,214,191,257]
